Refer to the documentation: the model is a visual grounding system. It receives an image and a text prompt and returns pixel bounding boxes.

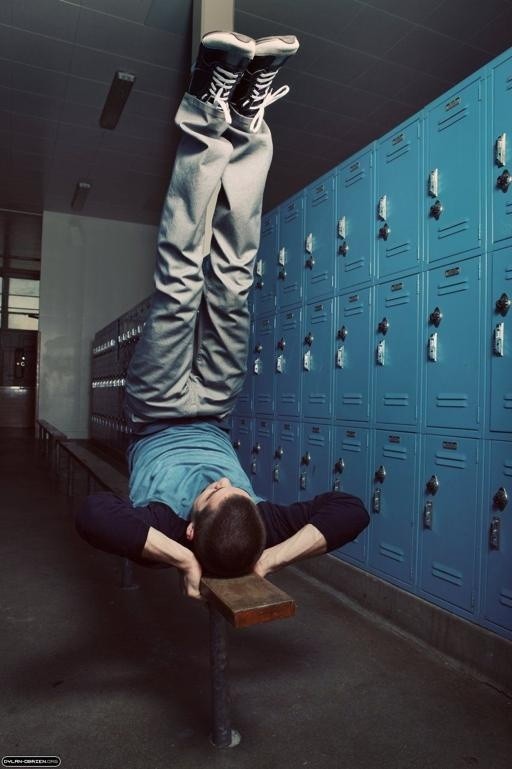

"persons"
[74,31,371,602]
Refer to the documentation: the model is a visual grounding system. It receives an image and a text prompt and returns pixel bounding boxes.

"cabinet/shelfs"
[89,45,508,637]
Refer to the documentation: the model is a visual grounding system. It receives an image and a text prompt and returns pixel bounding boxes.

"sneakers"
[188,31,300,119]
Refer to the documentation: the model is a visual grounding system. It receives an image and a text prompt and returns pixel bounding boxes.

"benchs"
[37,418,67,456]
[58,440,298,746]
